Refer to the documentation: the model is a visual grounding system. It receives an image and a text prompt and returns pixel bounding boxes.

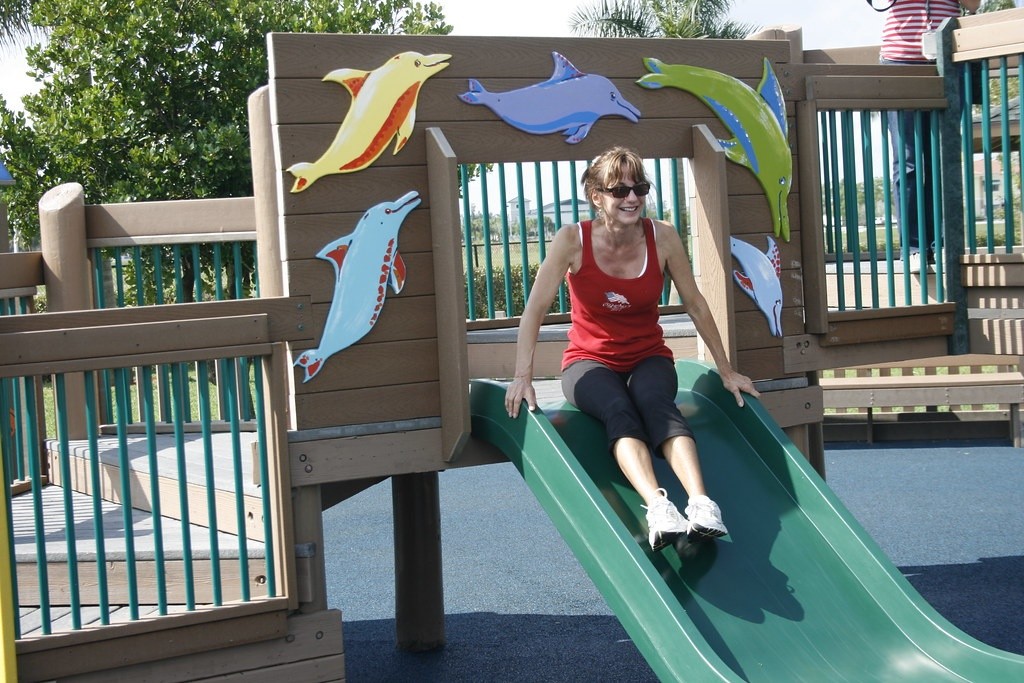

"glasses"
[598,182,651,199]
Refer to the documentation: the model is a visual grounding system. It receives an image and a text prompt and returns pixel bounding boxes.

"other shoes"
[929,248,946,266]
[909,251,929,275]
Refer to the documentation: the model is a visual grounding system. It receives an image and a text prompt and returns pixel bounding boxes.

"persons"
[878,0,983,274]
[505,144,760,553]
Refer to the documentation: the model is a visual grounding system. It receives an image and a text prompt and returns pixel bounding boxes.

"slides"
[467,359,1023,682]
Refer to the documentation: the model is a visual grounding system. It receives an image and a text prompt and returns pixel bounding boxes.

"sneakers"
[684,499,728,542]
[639,488,688,553]
